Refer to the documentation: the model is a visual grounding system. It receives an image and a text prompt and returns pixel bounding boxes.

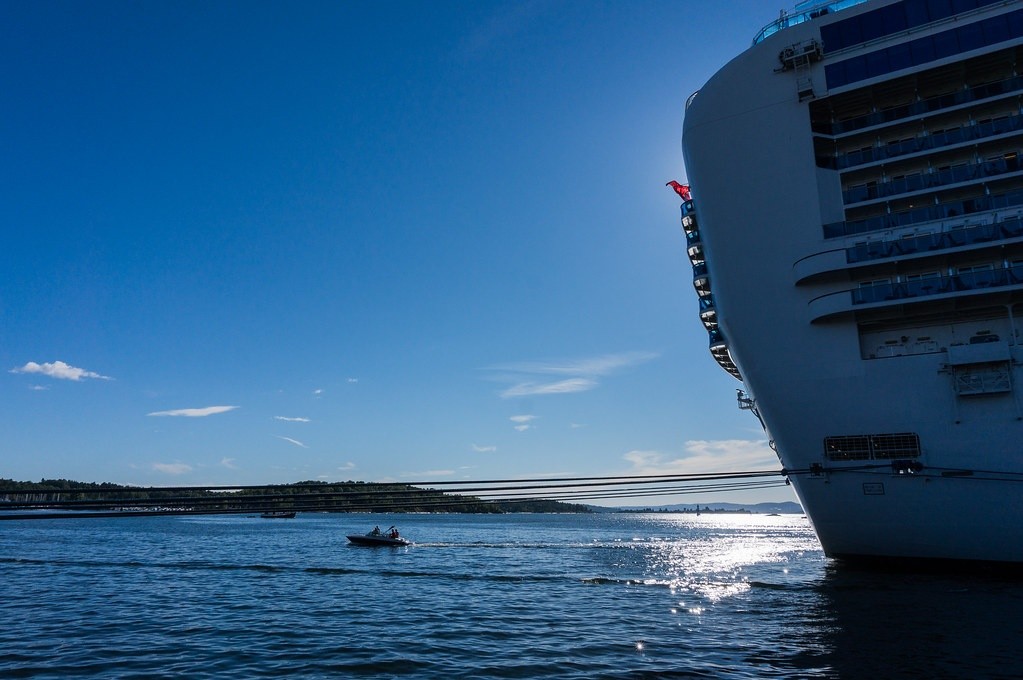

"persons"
[373,525,380,535]
[392,528,399,538]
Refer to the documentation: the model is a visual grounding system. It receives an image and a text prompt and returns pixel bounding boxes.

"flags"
[666,180,692,202]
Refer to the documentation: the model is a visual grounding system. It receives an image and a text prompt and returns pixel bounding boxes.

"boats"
[261,512,296,518]
[347,536,411,547]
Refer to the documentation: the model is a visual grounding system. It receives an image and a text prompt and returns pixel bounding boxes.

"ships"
[682,0,1023,585]
